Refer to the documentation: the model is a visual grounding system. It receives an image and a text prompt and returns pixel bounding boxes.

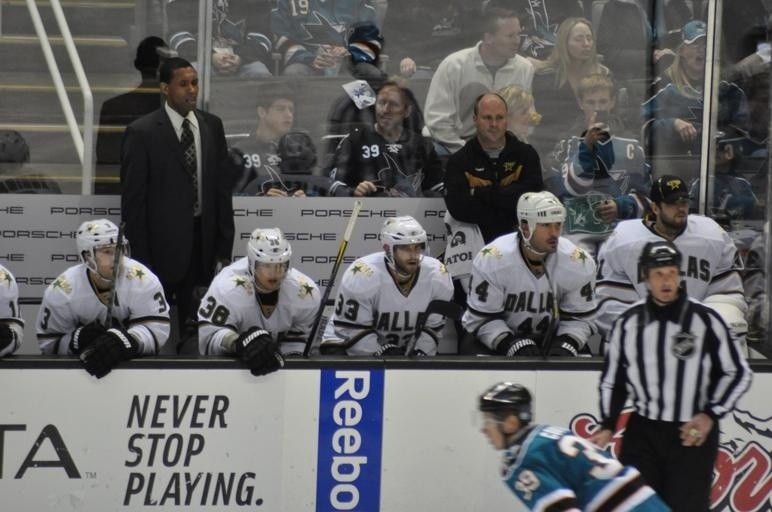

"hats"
[650,175,696,204]
[682,20,707,45]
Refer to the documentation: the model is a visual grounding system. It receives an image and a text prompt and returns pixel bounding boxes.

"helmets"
[480,382,533,422]
[639,241,682,266]
[275,133,315,154]
[77,219,128,274]
[517,190,566,242]
[247,227,292,279]
[378,215,426,264]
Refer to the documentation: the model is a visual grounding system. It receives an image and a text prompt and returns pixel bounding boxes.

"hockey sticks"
[404,299,461,355]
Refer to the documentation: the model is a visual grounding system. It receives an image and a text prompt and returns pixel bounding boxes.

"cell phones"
[595,109,608,131]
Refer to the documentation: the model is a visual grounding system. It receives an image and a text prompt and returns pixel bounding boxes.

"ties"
[179,120,200,215]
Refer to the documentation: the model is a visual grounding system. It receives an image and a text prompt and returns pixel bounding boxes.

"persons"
[424,6,538,165]
[228,82,319,198]
[388,0,479,76]
[328,85,446,199]
[333,215,455,357]
[260,131,341,199]
[640,19,750,174]
[544,74,652,239]
[462,191,597,357]
[0,264,25,359]
[196,227,322,376]
[120,57,236,333]
[531,16,619,151]
[35,218,170,378]
[686,130,758,221]
[166,0,272,79]
[595,175,749,340]
[93,36,171,198]
[480,0,586,76]
[476,380,672,512]
[327,21,442,173]
[586,240,753,512]
[446,93,544,246]
[273,1,385,80]
[0,129,61,194]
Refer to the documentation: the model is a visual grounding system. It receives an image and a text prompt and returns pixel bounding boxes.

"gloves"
[375,345,400,357]
[81,327,135,379]
[70,322,105,353]
[235,326,284,376]
[551,338,578,358]
[507,337,539,359]
